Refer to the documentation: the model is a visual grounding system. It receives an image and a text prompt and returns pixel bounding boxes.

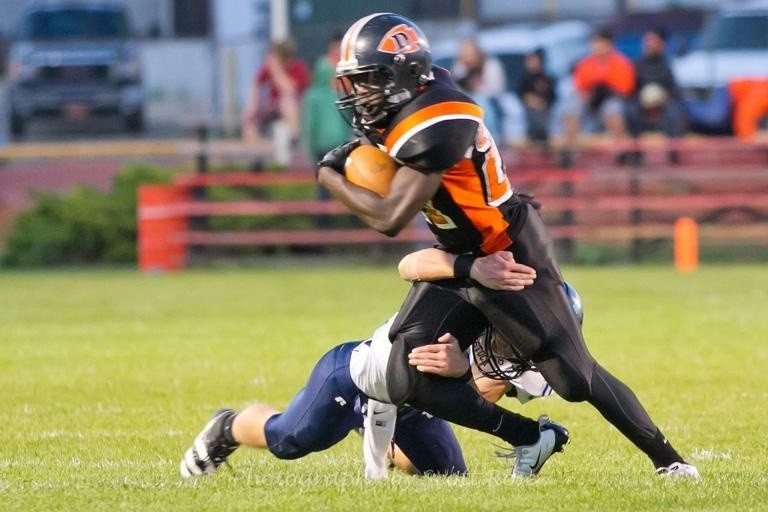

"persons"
[180,248,584,478]
[449,26,687,165]
[317,11,700,483]
[242,30,352,170]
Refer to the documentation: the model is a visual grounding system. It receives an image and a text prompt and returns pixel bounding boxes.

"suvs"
[5,2,143,133]
[672,3,767,130]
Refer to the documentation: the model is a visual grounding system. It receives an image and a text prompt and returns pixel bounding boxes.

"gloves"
[315,138,361,178]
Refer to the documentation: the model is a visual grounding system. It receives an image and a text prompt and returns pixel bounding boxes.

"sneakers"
[179,408,240,479]
[653,461,700,483]
[491,414,572,479]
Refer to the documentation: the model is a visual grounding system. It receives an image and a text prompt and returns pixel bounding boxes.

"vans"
[431,21,604,134]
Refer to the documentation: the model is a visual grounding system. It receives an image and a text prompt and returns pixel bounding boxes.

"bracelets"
[459,366,473,383]
[454,254,478,280]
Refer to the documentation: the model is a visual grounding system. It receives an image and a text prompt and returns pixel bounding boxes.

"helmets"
[334,12,432,141]
[470,278,584,379]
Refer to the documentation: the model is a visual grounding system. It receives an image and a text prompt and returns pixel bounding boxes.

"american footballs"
[343,145,397,197]
